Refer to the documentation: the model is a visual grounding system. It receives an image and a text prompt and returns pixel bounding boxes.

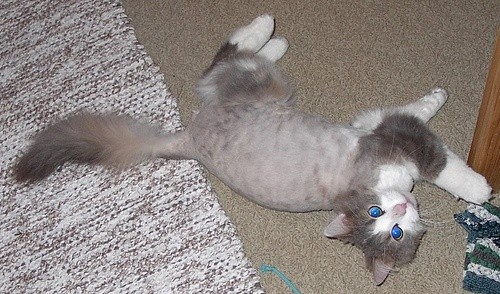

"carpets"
[0,0,263,293]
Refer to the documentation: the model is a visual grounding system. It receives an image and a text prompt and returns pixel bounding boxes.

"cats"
[10,14,493,285]
